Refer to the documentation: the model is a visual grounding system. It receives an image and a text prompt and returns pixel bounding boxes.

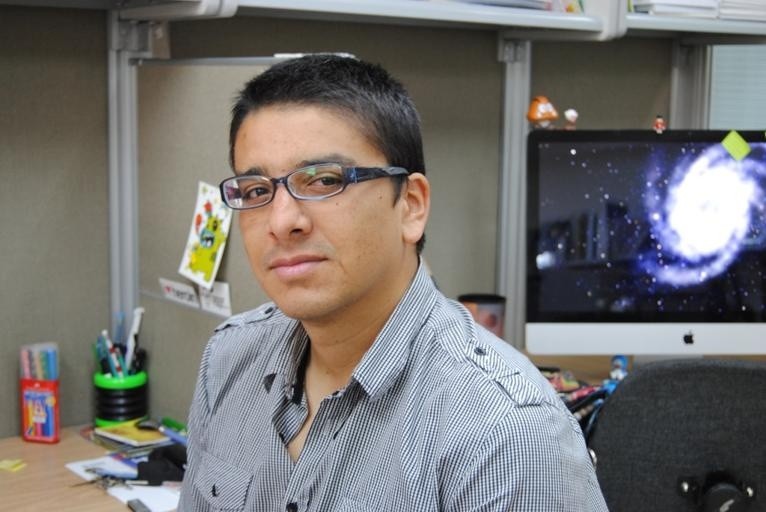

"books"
[93,417,172,448]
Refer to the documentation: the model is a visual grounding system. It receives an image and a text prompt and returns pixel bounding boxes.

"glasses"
[219,161,408,211]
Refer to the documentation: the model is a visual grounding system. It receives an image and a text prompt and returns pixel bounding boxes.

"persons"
[177,52,611,512]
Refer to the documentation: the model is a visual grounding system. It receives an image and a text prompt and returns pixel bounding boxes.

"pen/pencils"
[92,306,146,378]
[18,344,57,438]
[158,426,187,445]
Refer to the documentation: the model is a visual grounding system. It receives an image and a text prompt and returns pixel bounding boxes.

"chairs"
[588,358,765,512]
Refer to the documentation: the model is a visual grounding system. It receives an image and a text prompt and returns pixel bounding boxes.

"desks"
[0,419,183,512]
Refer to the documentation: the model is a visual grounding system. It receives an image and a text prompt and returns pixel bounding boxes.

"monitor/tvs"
[524,129,766,357]
